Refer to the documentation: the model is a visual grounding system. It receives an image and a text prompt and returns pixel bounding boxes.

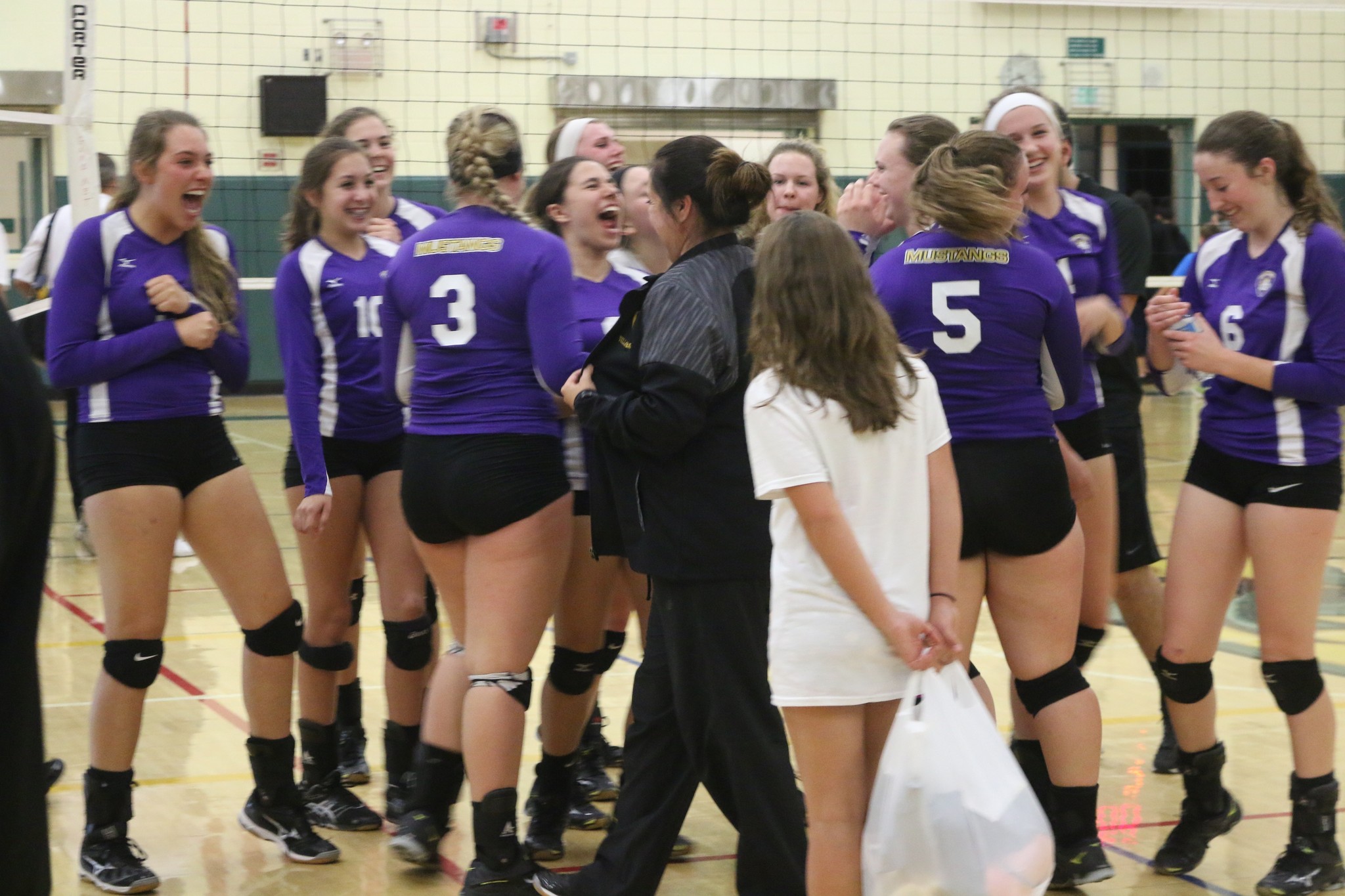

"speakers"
[261,75,325,134]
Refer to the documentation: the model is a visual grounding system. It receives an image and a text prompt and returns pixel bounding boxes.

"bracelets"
[930,591,957,603]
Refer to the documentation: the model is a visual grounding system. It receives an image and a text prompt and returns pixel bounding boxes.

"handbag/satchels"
[859,645,1057,896]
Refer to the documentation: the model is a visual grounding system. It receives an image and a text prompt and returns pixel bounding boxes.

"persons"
[1144,109,1345,896]
[1,86,1224,896]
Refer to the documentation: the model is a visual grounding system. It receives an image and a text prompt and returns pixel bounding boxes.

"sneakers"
[525,776,611,829]
[459,842,550,896]
[576,744,619,802]
[239,782,339,864]
[295,769,384,830]
[1155,788,1240,875]
[1153,705,1180,774]
[604,811,693,855]
[523,806,563,859]
[529,866,590,896]
[382,777,448,865]
[336,721,372,785]
[537,707,625,767]
[1255,825,1344,895]
[1050,828,1112,888]
[81,817,160,893]
